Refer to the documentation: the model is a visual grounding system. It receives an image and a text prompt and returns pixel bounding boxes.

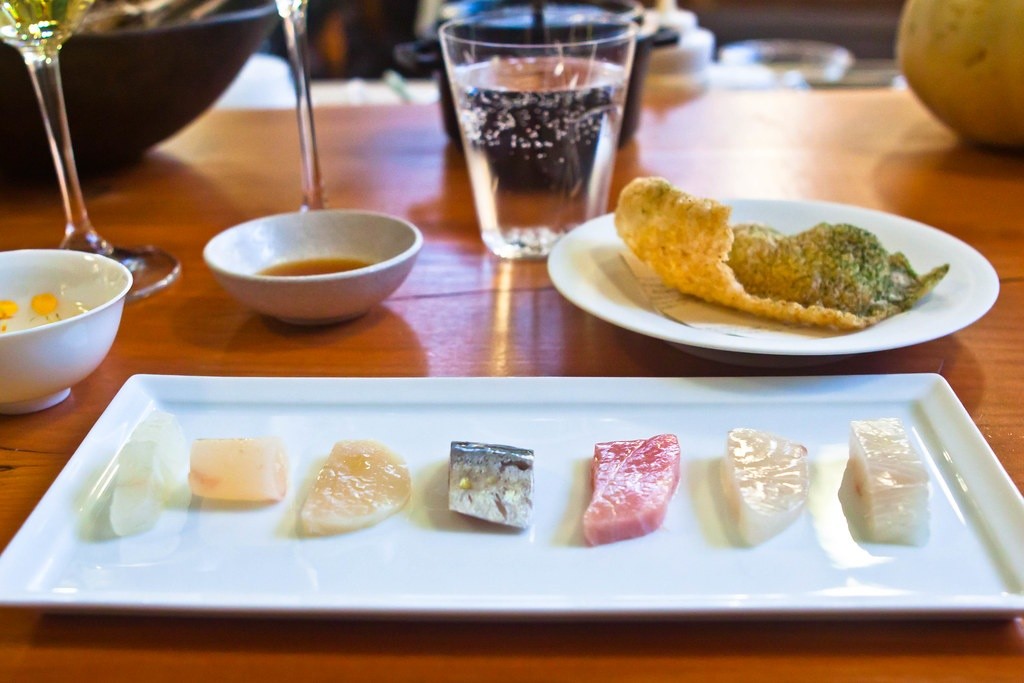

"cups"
[438,11,640,259]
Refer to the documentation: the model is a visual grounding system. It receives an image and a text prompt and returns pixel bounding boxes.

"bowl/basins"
[0,248,134,415]
[201,209,425,326]
[0,2,283,195]
[394,9,680,154]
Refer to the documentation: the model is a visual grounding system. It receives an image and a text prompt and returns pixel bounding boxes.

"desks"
[0,77,1024,683]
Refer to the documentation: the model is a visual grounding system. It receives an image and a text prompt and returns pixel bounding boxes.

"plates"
[0,371,1024,623]
[547,196,1000,369]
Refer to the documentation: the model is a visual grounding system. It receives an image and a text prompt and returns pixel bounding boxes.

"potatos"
[896,0,1024,150]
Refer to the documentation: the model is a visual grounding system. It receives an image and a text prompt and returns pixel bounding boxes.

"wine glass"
[0,0,182,304]
